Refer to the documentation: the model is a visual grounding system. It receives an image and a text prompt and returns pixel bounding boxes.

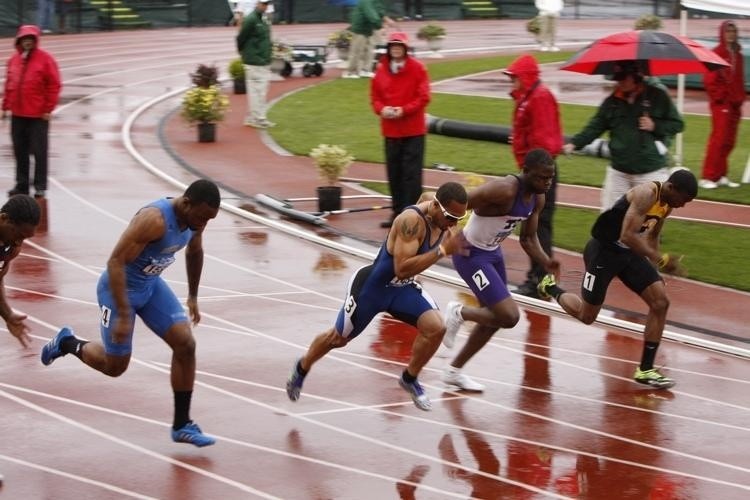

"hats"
[502,69,515,78]
[605,61,640,81]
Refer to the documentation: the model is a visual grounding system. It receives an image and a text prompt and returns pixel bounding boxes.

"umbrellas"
[559,32,733,116]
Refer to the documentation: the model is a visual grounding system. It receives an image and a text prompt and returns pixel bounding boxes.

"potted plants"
[416,24,448,50]
[329,30,353,60]
[270,39,293,74]
[179,85,231,142]
[230,60,246,95]
[310,144,356,211]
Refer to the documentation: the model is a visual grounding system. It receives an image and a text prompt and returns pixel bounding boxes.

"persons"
[40,180,219,447]
[536,169,699,387]
[562,65,686,212]
[2,196,39,349]
[502,55,563,299]
[234,2,274,130]
[416,146,562,393]
[339,2,398,81]
[535,0,565,50]
[0,25,65,196]
[698,22,746,190]
[286,181,474,411]
[367,32,431,228]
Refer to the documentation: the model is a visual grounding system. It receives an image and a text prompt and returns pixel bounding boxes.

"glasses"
[432,195,468,220]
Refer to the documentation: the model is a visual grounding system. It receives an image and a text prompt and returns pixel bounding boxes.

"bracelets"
[659,254,669,271]
[436,245,447,263]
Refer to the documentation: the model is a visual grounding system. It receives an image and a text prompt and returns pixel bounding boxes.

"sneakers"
[171,420,215,448]
[245,116,276,129]
[697,179,719,189]
[34,189,44,196]
[9,186,29,196]
[716,176,741,188]
[42,328,74,365]
[382,220,392,228]
